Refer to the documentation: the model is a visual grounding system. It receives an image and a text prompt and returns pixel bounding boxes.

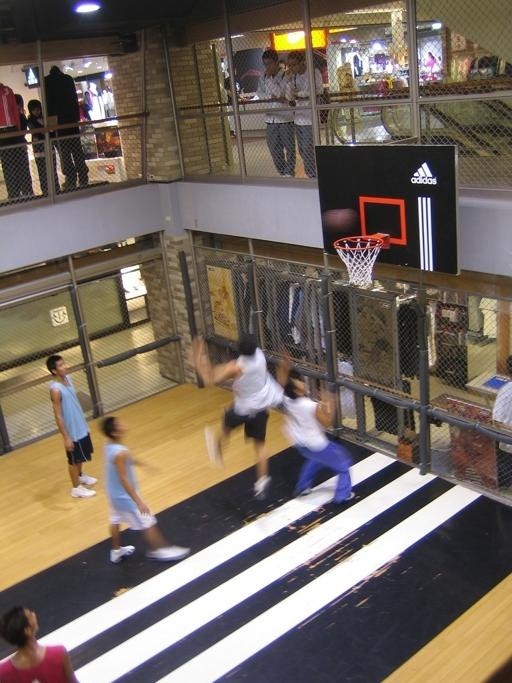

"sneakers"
[152,545,190,560]
[71,485,96,498]
[110,544,135,563]
[254,476,271,497]
[79,474,97,484]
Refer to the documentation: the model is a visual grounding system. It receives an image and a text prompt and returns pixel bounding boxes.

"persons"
[0,604,84,683]
[44,354,99,498]
[99,414,192,565]
[8,91,29,158]
[423,51,438,81]
[100,89,112,118]
[278,49,325,178]
[82,86,97,111]
[1,81,34,200]
[41,64,90,190]
[489,354,512,487]
[238,47,298,177]
[188,326,284,502]
[24,98,64,197]
[270,344,357,504]
[80,92,92,121]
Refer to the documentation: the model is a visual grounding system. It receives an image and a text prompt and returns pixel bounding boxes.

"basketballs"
[324,206,355,231]
